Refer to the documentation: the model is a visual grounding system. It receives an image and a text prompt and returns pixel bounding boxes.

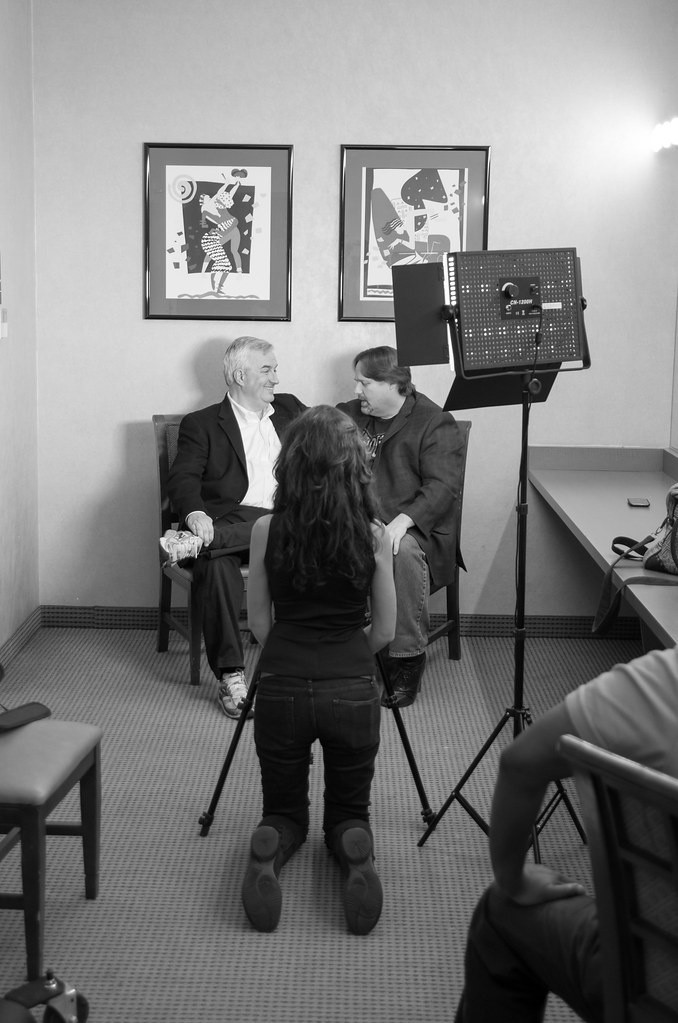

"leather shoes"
[380,651,426,707]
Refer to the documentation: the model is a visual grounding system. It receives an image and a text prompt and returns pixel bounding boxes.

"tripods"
[198,654,436,837]
[417,372,587,869]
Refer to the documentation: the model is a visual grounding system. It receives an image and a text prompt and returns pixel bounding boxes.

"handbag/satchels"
[591,483,678,632]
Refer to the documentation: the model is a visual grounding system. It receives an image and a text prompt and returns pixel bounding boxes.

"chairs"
[426,420,472,661]
[153,414,260,684]
[555,733,678,1023]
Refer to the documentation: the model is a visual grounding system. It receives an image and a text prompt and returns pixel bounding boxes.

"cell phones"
[628,498,650,507]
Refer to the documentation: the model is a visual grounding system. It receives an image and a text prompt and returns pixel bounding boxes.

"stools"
[0,718,103,982]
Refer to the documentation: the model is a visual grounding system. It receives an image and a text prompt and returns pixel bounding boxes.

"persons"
[241,403,398,936]
[159,337,311,719]
[335,346,469,709]
[453,640,678,1023]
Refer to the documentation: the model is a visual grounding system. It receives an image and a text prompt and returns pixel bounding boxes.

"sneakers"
[159,529,203,567]
[218,668,255,718]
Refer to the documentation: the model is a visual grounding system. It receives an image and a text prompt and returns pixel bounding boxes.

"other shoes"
[336,820,382,936]
[240,817,285,932]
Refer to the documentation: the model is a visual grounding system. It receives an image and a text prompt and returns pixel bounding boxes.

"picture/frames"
[337,144,490,322]
[143,143,292,322]
